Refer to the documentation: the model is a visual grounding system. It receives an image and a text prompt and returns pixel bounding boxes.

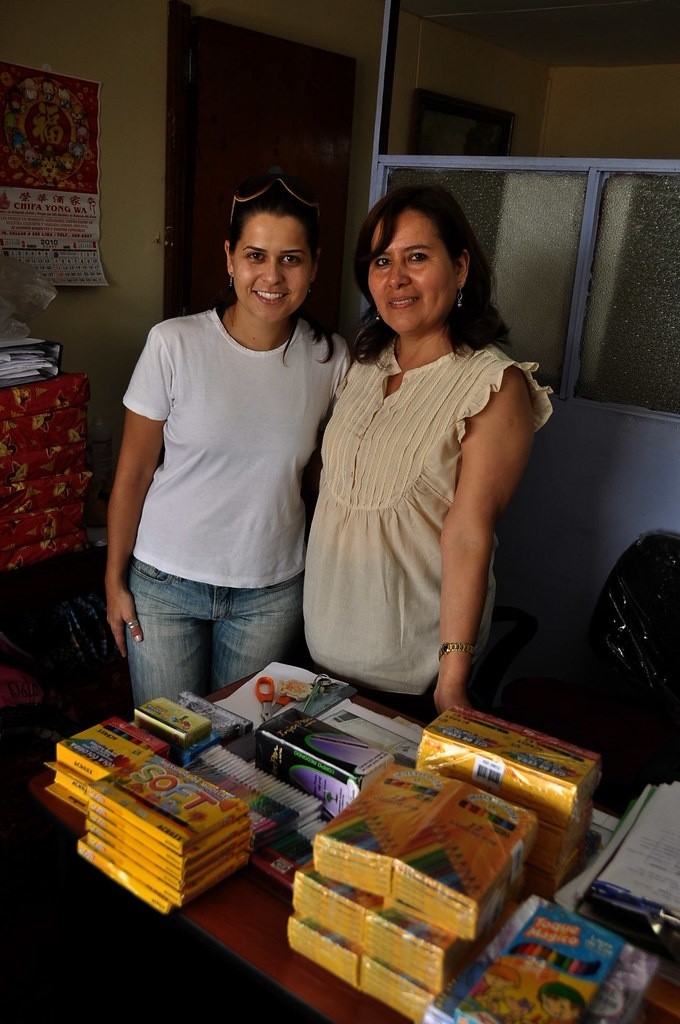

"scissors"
[302,673,338,714]
[256,678,296,722]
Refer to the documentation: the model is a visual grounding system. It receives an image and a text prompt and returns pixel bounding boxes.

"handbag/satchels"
[54,595,117,675]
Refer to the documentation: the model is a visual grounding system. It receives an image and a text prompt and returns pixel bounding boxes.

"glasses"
[230,174,322,234]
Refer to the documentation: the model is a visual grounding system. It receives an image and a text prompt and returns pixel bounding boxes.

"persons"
[104,173,352,712]
[302,182,553,715]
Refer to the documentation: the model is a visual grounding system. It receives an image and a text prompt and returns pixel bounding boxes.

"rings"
[128,620,138,629]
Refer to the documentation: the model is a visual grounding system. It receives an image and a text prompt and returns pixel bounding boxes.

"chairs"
[473,532,680,812]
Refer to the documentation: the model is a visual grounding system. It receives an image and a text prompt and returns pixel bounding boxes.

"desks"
[27,670,680,1024]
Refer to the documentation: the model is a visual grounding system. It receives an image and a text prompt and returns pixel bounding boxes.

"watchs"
[438,643,477,660]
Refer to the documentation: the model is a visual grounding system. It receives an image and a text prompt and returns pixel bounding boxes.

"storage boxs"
[44,697,253,915]
[0,368,92,574]
[287,704,660,1024]
[255,708,392,822]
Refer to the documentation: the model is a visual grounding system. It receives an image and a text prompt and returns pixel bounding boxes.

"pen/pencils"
[514,942,597,978]
[186,744,327,886]
[399,817,479,897]
[323,798,397,856]
